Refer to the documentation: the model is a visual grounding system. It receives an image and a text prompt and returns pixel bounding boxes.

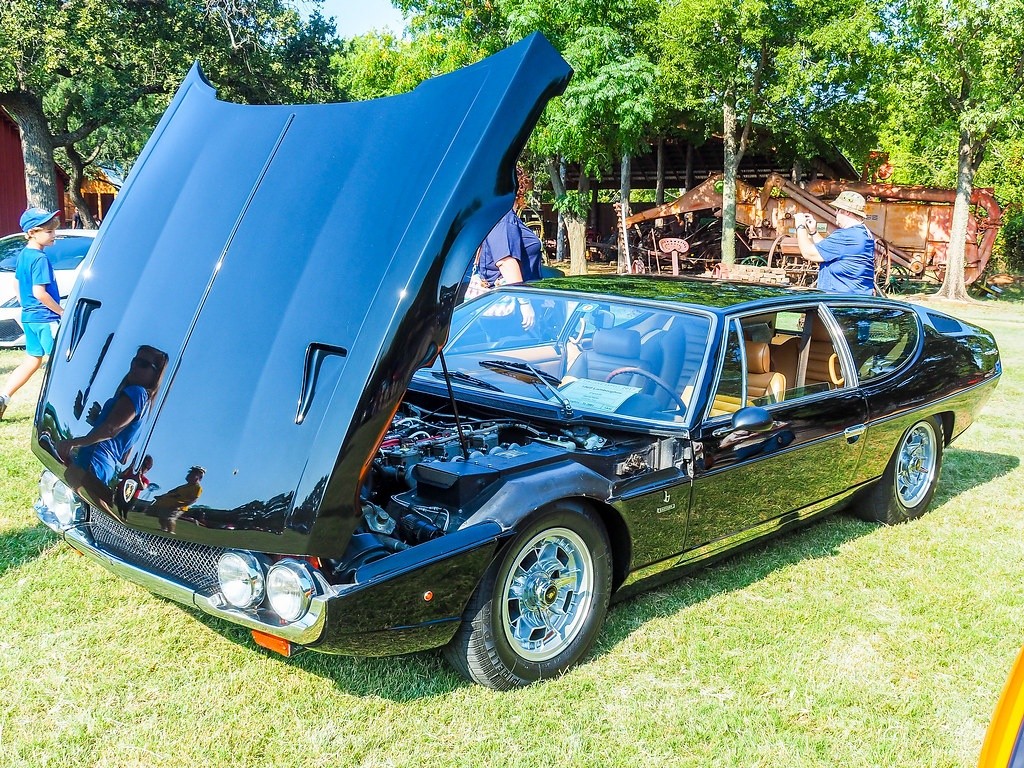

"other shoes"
[158,528,168,532]
[168,531,176,535]
[0,396,7,422]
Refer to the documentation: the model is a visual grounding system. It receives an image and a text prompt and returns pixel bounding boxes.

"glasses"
[131,357,159,371]
[188,471,197,475]
[836,207,841,212]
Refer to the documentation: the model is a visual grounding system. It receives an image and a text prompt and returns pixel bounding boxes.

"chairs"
[675,341,787,423]
[556,328,652,390]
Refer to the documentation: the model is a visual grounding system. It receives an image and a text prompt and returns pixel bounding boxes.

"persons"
[0,207,64,421]
[474,164,543,344]
[794,190,874,345]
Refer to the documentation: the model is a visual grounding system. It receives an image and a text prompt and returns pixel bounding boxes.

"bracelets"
[796,224,807,230]
[809,231,817,236]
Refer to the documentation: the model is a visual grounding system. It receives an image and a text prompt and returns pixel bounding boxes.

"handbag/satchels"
[464,274,516,318]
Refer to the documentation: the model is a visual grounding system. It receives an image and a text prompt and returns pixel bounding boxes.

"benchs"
[642,313,889,400]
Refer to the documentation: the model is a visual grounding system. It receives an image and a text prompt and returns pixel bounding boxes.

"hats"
[829,191,867,219]
[20,208,61,232]
[190,466,205,476]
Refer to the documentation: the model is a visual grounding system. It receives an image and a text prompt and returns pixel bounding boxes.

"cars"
[0,228,99,348]
[32,28,1005,692]
